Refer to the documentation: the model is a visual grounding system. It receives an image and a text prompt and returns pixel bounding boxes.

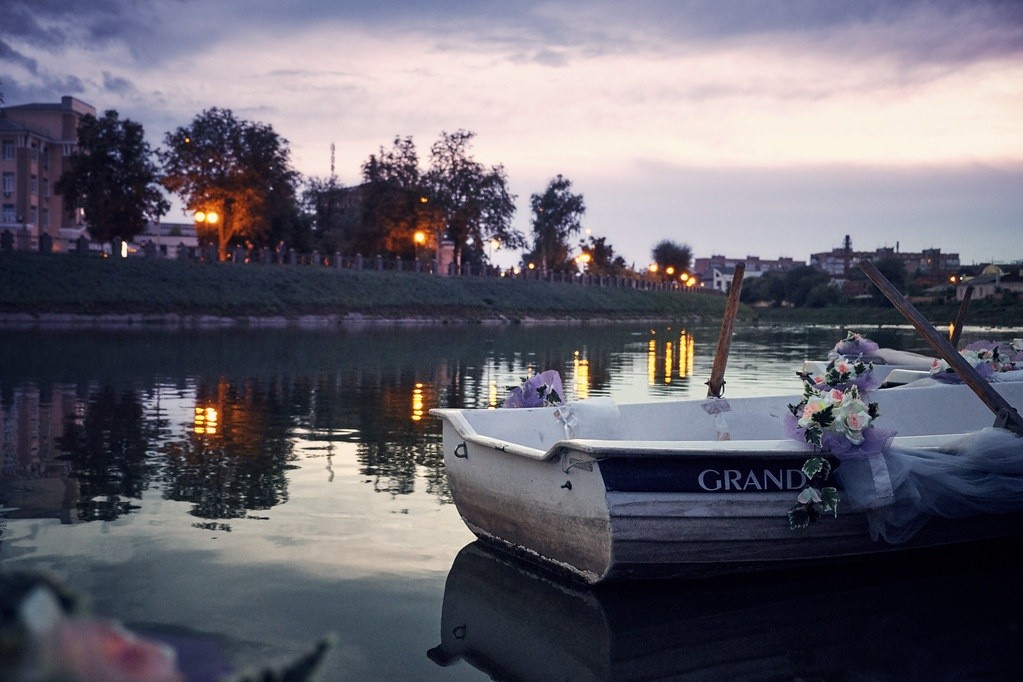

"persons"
[672,277,677,285]
[244,239,254,264]
[275,240,287,265]
[510,266,514,277]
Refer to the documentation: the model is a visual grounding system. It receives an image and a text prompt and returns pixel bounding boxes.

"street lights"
[196,211,218,260]
[649,263,658,281]
[576,255,591,273]
[412,231,425,268]
[667,265,674,286]
[681,273,697,288]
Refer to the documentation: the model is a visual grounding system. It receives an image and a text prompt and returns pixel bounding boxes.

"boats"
[798,358,935,391]
[430,375,1023,585]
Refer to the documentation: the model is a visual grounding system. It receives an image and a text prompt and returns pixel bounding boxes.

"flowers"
[487,365,562,408]
[930,345,1017,374]
[788,353,880,532]
[840,330,868,347]
